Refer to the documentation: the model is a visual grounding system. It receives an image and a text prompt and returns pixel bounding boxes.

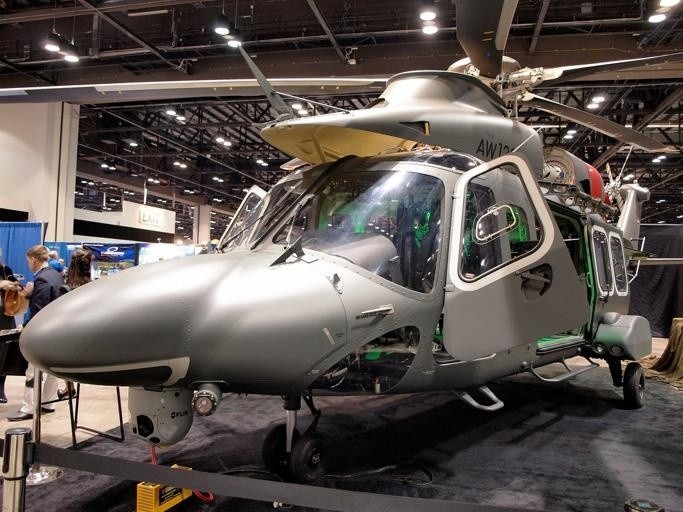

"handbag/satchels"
[3,285,28,316]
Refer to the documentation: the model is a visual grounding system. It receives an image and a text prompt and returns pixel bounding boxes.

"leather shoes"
[7,412,33,421]
[41,407,53,413]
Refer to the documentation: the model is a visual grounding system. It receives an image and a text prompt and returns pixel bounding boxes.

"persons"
[0,248,23,405]
[8,244,64,421]
[65,248,92,292]
[58,259,68,270]
[57,378,76,401]
[91,254,96,269]
[47,250,64,271]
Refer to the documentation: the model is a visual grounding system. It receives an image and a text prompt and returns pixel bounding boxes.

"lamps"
[64,0,80,63]
[214,0,229,35]
[227,0,241,47]
[44,0,61,53]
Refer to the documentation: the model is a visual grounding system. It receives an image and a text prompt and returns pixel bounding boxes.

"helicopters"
[0,0,683,485]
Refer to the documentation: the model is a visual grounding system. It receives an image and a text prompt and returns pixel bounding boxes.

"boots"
[0,376,7,403]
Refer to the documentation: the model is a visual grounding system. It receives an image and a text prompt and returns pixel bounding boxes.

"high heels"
[57,390,75,398]
